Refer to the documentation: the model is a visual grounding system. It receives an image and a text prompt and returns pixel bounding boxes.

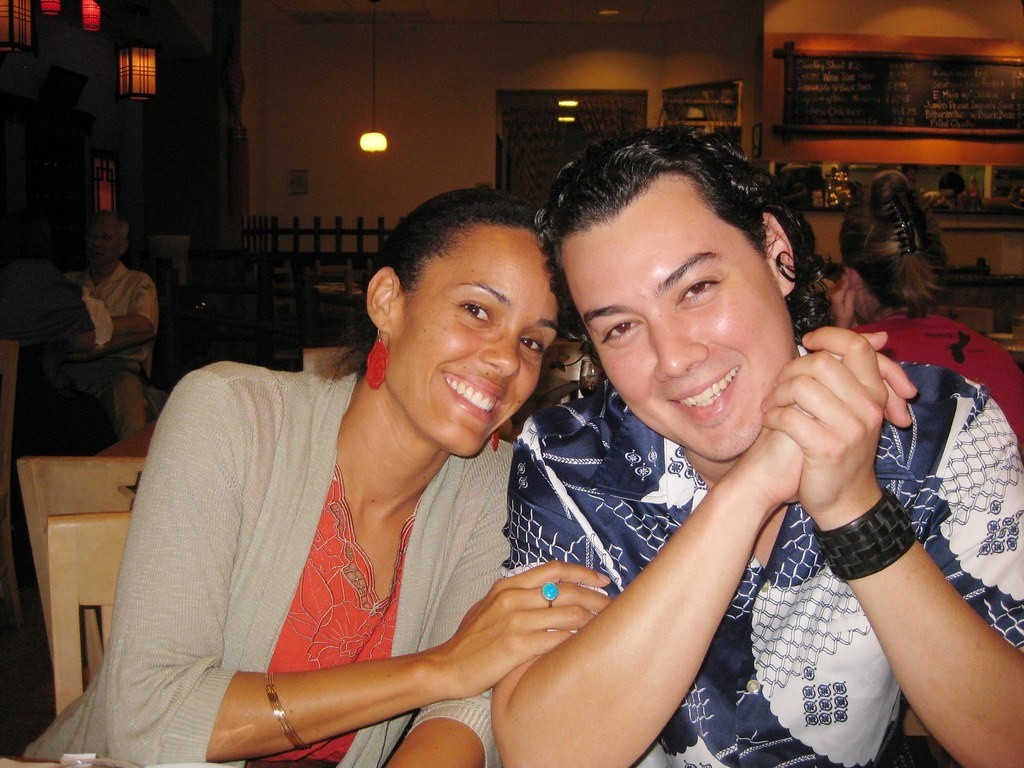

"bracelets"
[812,486,917,581]
[264,672,311,750]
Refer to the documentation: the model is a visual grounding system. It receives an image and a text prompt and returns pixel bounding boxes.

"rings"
[542,582,559,608]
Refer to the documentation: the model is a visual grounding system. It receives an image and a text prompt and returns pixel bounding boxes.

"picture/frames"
[290,169,309,195]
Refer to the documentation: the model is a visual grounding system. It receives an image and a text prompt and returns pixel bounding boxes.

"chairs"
[19,455,148,692]
[42,511,134,719]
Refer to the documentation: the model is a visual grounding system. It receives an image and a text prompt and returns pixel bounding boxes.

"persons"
[23,186,610,768]
[925,172,966,202]
[0,208,119,454]
[827,170,1024,459]
[492,124,1024,768]
[62,210,159,437]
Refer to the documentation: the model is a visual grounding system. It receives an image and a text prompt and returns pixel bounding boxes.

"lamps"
[359,0,388,153]
